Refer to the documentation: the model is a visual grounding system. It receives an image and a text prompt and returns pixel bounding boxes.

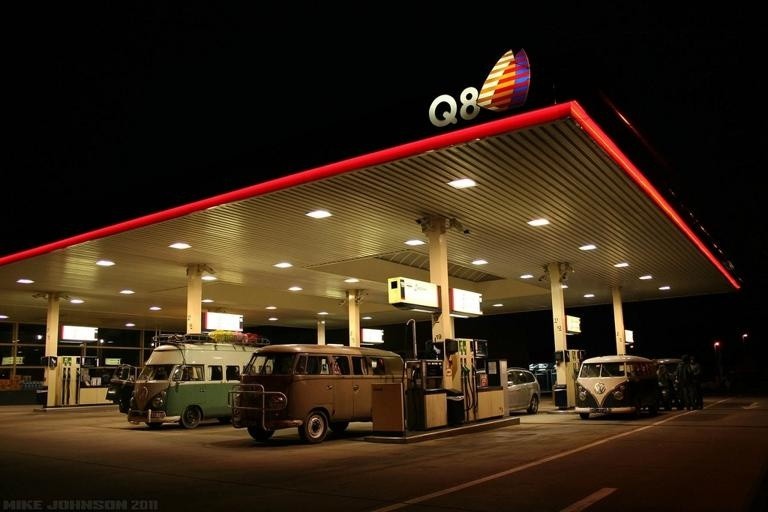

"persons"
[655,353,706,412]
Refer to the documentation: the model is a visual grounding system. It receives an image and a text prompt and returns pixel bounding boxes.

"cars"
[507,367,541,413]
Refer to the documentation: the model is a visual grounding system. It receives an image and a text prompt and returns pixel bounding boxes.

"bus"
[128,331,275,429]
[574,356,703,419]
[226,345,408,444]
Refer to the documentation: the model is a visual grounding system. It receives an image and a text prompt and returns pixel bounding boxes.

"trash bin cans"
[447,388,465,425]
[555,384,567,406]
[36,386,48,404]
